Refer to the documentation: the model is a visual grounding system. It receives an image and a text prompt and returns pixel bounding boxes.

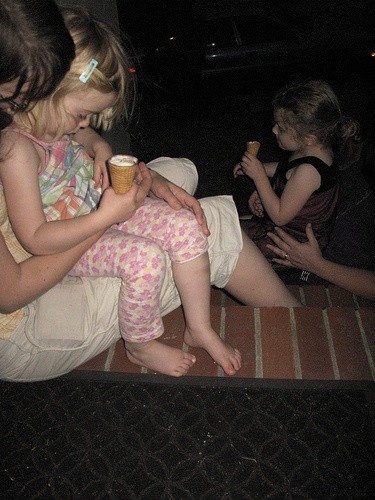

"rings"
[283,252,288,261]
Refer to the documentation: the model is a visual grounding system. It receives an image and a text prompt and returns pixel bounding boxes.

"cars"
[158,2,298,74]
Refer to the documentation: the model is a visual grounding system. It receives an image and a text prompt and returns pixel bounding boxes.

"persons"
[0,6,241,376]
[236,154,374,304]
[0,0,305,383]
[233,80,360,276]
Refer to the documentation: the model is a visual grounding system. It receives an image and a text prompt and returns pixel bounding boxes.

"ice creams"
[247,141,260,158]
[107,154,138,193]
